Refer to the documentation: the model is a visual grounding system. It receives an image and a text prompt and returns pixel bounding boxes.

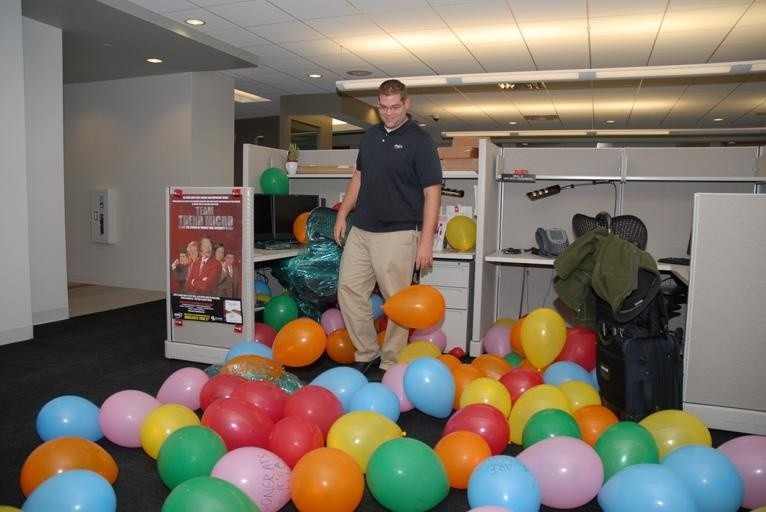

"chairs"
[272,205,354,317]
[569,213,689,319]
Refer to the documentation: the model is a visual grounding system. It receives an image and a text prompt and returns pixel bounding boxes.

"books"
[261,168,289,195]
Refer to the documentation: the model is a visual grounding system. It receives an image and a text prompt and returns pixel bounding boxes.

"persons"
[172,236,237,301]
[333,79,443,378]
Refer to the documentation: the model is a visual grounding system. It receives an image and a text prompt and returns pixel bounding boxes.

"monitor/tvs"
[272,194,321,240]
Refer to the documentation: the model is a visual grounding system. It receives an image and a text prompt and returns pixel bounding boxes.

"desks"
[252,245,474,361]
[485,248,690,287]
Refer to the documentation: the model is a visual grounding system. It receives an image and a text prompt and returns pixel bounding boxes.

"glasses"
[378,104,401,111]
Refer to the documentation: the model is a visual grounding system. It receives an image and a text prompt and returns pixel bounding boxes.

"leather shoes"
[352,360,372,373]
[367,367,387,382]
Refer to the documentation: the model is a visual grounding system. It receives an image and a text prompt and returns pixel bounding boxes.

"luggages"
[590,325,684,428]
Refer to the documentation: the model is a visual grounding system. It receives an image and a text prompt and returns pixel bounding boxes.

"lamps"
[333,58,766,95]
[525,178,619,214]
[441,179,464,198]
[438,125,766,139]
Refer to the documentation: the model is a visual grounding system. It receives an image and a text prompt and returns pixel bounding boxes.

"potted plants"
[283,142,300,176]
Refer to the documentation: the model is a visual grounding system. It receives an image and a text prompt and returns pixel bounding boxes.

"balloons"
[331,202,356,213]
[447,215,477,252]
[382,285,446,329]
[293,212,311,243]
[23,307,764,512]
[256,280,446,368]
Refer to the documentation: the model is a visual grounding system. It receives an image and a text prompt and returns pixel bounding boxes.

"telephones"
[535,228,570,256]
